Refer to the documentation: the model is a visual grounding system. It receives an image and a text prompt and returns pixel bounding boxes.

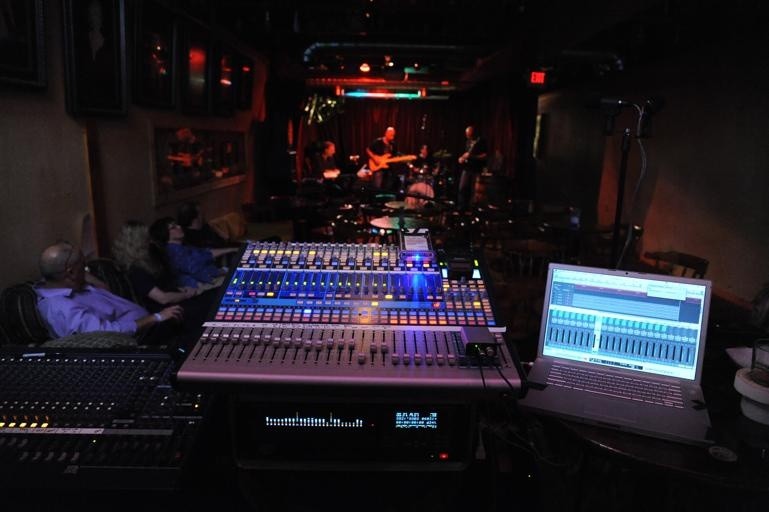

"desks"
[503,309,768,510]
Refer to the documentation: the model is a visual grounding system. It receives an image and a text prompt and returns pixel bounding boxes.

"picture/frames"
[1,0,254,119]
[146,119,251,209]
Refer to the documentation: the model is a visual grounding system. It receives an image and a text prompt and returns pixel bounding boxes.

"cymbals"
[385,201,424,211]
[370,218,434,230]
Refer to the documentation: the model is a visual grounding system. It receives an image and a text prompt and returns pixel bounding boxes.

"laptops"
[522,262,714,446]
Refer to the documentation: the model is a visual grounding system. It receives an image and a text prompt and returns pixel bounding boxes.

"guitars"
[368,153,417,172]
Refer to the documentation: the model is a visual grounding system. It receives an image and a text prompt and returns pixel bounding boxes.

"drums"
[409,180,433,198]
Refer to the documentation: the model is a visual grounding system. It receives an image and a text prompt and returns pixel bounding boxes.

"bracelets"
[153,314,164,323]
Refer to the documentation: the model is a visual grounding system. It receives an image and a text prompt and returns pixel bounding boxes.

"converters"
[460,326,497,355]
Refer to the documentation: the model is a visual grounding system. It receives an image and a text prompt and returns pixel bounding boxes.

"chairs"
[0,207,296,359]
[445,209,769,361]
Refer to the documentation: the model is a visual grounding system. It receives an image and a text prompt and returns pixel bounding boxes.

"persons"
[314,138,336,178]
[412,142,440,176]
[31,239,194,349]
[366,123,401,189]
[110,224,216,329]
[150,215,240,300]
[176,200,232,274]
[455,126,489,210]
